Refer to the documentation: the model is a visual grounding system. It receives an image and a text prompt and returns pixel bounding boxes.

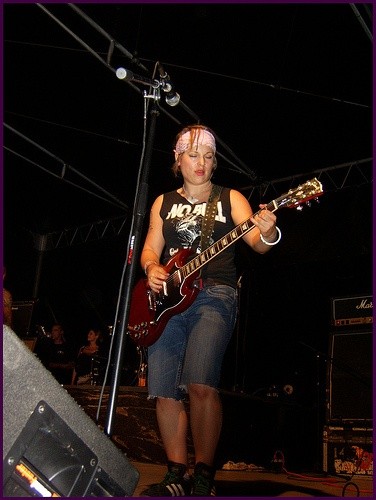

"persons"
[141,124,276,497]
[33,325,108,386]
[3,266,12,325]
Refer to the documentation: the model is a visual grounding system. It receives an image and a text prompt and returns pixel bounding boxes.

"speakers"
[0,323,140,497]
[323,330,375,425]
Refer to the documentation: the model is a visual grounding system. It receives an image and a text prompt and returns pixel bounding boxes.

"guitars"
[127,176,324,347]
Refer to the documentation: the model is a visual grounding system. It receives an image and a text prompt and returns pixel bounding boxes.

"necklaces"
[183,182,211,199]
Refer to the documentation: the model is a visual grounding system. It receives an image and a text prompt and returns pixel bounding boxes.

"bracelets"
[145,261,158,275]
[260,226,282,245]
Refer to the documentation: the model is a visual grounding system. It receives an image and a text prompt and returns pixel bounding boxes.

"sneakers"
[140,460,193,497]
[190,463,216,496]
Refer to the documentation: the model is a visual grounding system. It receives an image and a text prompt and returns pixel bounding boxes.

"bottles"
[138,363,146,387]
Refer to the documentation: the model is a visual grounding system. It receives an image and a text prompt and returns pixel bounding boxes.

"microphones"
[158,66,180,106]
